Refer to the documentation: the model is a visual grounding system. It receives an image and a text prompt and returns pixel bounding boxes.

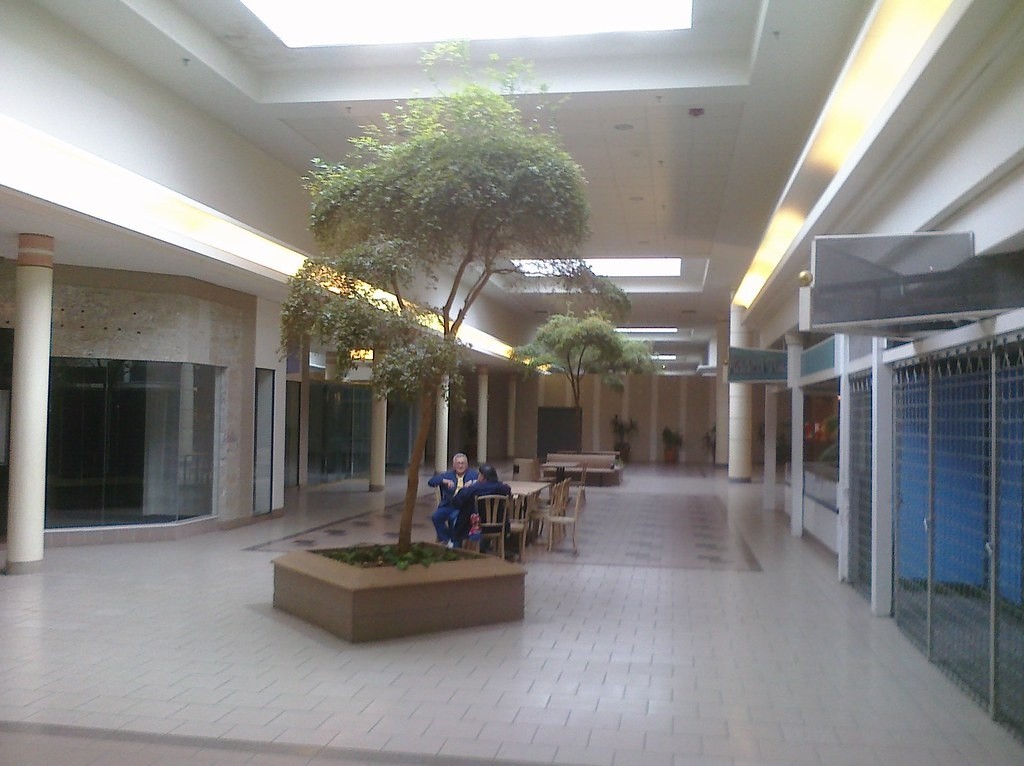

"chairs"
[535,481,563,538]
[528,484,557,540]
[466,494,509,560]
[572,462,587,495]
[507,492,533,562]
[568,462,586,494]
[538,478,567,536]
[545,486,587,555]
[541,477,571,508]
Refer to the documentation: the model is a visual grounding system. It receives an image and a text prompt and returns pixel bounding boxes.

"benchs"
[545,454,616,474]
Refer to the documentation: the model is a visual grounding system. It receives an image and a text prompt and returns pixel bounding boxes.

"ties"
[455,475,463,498]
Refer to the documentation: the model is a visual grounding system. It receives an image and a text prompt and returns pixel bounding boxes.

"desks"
[499,480,551,546]
[541,460,581,483]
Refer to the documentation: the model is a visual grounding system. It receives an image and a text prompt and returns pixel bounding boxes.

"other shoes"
[438,539,454,550]
[508,553,521,562]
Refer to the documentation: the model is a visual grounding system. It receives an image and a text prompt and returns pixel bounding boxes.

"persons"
[448,464,512,554]
[427,453,480,544]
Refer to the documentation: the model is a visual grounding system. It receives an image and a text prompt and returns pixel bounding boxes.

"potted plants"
[700,424,716,462]
[610,414,638,463]
[663,425,683,463]
